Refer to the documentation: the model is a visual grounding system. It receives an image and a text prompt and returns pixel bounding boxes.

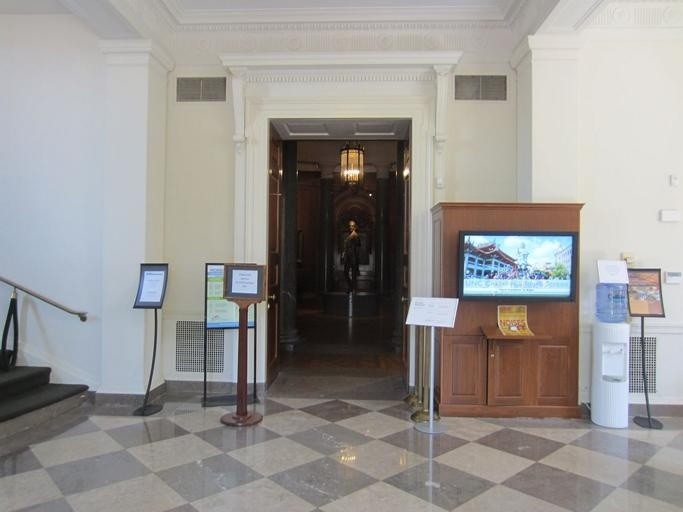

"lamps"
[340,142,365,188]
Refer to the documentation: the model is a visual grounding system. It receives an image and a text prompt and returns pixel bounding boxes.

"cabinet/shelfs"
[429,201,581,418]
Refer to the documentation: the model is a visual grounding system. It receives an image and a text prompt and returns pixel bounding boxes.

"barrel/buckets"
[594,283,628,324]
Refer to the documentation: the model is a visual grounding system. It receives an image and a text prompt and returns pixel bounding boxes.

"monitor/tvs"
[456,230,578,302]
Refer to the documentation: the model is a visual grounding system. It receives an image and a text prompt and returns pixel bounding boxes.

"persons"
[340,221,361,301]
